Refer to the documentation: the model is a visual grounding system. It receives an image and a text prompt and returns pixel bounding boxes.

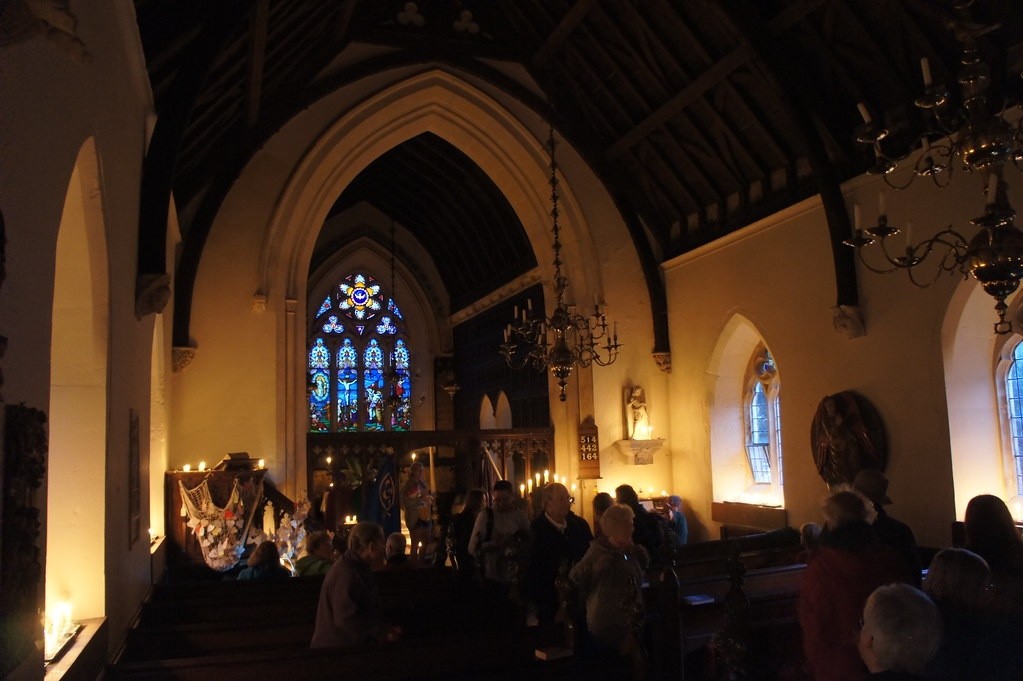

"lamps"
[500,47,623,400]
[372,210,424,422]
[519,469,575,519]
[843,0,1023,334]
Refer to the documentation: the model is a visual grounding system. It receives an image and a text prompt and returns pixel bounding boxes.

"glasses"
[493,498,508,503]
[553,497,573,505]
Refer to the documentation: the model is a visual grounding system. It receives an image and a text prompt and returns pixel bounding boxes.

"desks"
[165,469,267,564]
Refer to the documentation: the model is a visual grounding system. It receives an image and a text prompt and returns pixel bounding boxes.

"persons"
[146,461,1023,681]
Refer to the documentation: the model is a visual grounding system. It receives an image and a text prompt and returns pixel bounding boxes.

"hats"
[853,470,893,505]
[665,496,679,507]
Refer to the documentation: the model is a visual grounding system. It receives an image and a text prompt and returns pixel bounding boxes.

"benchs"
[106,528,808,681]
